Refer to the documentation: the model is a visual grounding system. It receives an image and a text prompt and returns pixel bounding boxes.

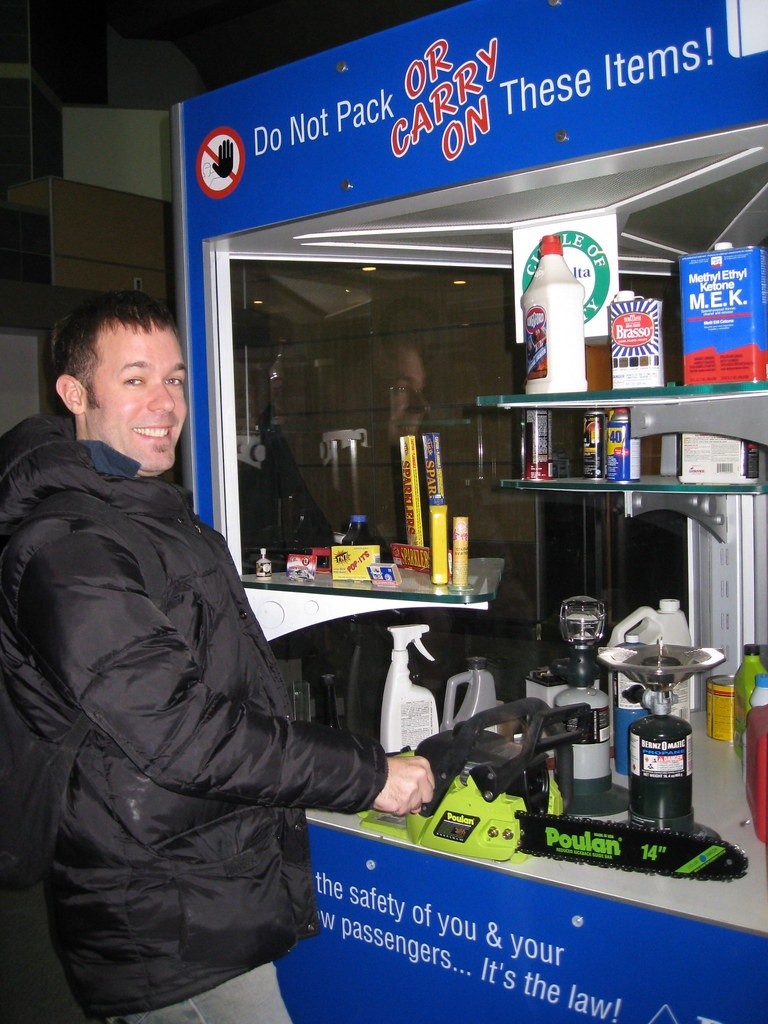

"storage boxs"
[675,432,759,485]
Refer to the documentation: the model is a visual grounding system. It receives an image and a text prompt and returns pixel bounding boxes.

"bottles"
[342,515,373,546]
[439,658,497,737]
[612,635,652,773]
[521,235,588,395]
[523,408,554,480]
[583,408,641,484]
[322,674,339,731]
[734,644,768,844]
[605,598,689,748]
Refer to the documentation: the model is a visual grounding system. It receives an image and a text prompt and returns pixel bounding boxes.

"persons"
[0,289,434,1024]
[230,305,544,703]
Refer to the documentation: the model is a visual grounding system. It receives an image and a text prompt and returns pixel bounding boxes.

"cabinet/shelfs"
[475,378,768,499]
